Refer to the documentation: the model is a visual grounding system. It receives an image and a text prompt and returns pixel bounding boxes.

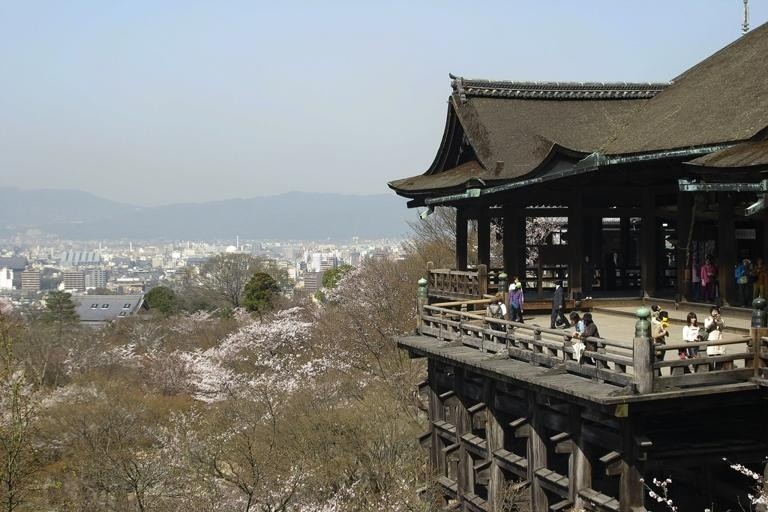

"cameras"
[711,313,718,317]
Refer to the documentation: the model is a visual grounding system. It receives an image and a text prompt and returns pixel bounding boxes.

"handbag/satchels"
[697,327,709,341]
[555,318,564,326]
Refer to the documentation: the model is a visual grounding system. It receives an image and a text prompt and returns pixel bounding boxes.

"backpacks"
[489,307,506,331]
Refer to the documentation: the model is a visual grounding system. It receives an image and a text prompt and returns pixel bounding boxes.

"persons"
[684,240,768,307]
[640,300,724,378]
[478,273,613,372]
